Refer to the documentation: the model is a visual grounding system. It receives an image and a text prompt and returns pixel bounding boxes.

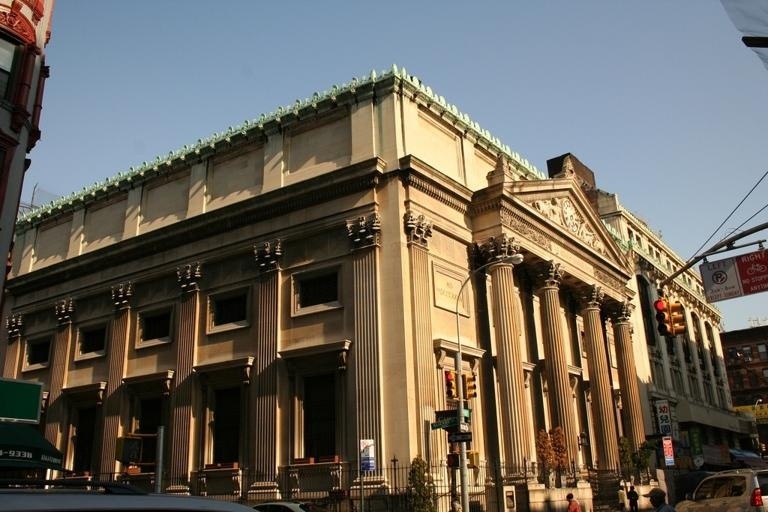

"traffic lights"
[654,299,686,338]
[445,371,457,398]
[462,374,477,400]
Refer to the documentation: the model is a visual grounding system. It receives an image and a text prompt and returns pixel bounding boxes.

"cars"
[254,501,331,512]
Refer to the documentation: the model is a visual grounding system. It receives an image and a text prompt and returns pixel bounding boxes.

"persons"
[643,488,675,511]
[627,485,639,512]
[566,492,581,512]
[618,485,627,512]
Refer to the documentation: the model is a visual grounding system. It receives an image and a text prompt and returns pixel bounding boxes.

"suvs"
[673,468,768,512]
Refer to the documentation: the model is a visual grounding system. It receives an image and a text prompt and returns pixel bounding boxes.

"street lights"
[456,254,523,511]
[755,399,762,425]
